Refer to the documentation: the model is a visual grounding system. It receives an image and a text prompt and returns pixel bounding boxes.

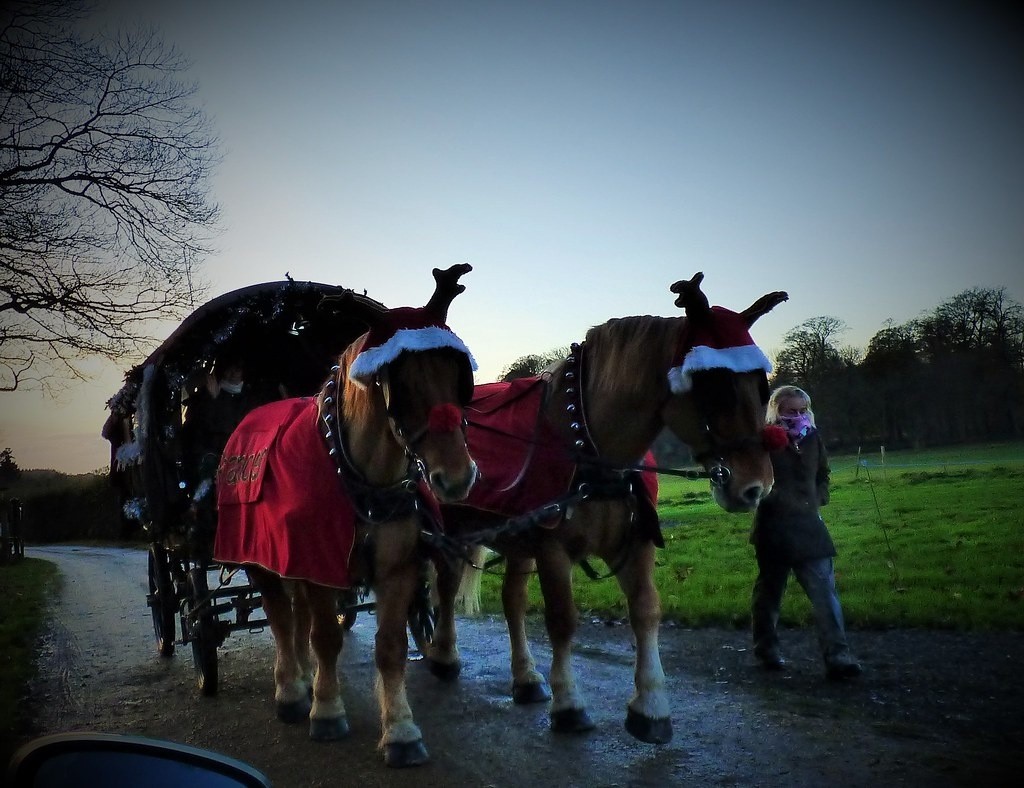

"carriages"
[100,262,789,766]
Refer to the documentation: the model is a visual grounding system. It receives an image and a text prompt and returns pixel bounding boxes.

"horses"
[205,302,478,770]
[424,290,790,746]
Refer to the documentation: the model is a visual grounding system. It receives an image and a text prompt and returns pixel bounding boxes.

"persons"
[176,360,260,461]
[749,385,862,683]
